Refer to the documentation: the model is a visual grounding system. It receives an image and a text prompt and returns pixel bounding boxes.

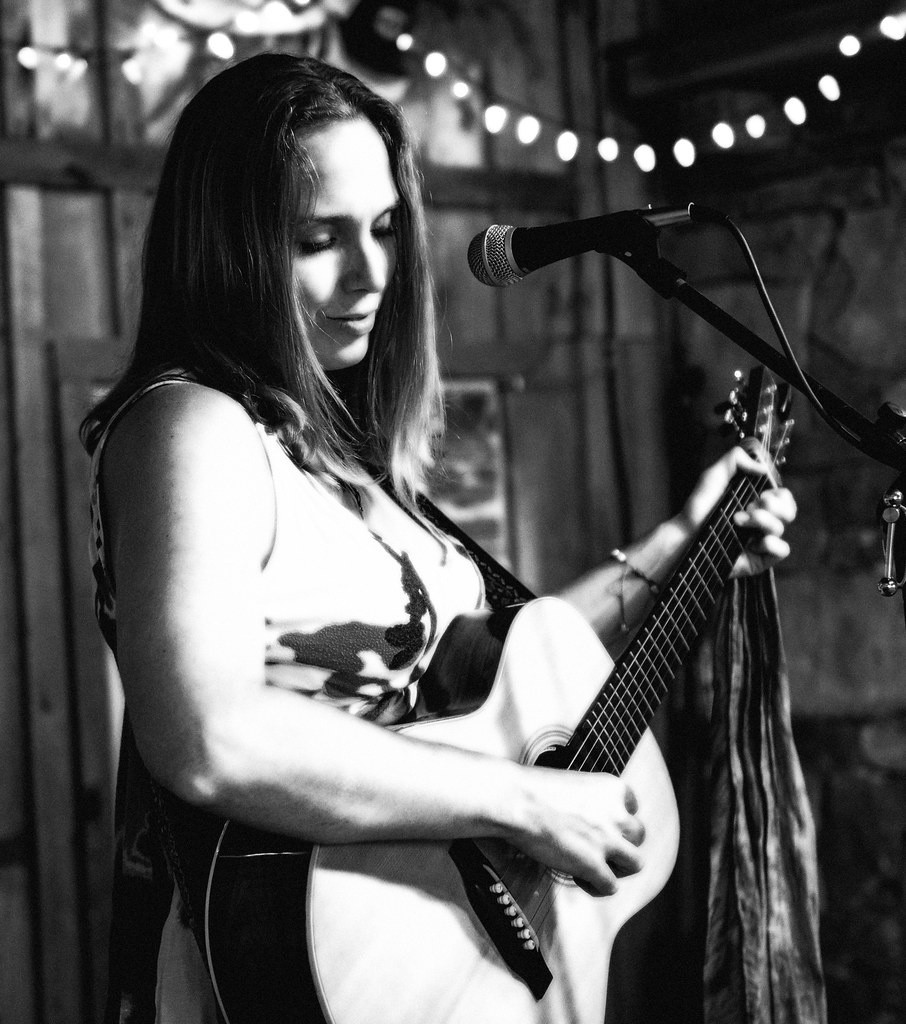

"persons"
[76,52,796,1024]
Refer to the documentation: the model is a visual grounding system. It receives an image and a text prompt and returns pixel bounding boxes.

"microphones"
[466,200,697,287]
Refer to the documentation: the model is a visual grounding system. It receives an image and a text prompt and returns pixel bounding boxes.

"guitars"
[206,353,801,1022]
